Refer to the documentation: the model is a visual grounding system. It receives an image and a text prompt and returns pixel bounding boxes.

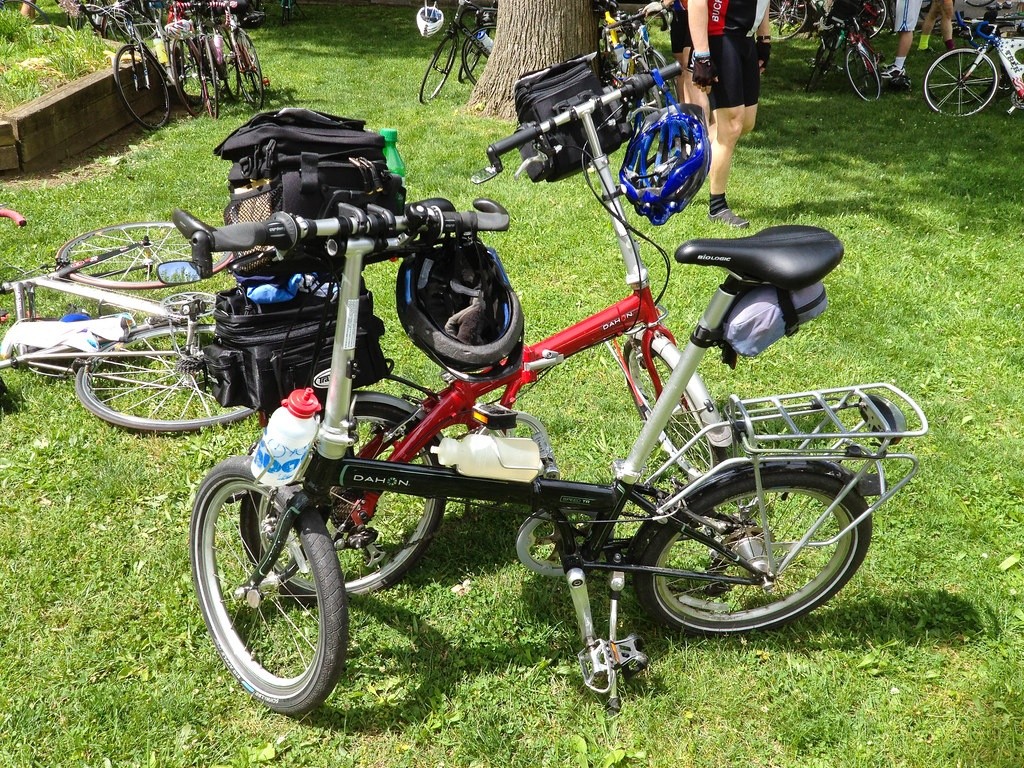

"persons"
[878,0,923,79]
[642,0,771,229]
[917,0,958,53]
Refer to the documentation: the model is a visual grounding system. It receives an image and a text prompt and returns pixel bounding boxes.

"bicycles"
[1,207,258,431]
[418,0,679,116]
[768,0,1024,118]
[79,1,269,130]
[170,51,929,724]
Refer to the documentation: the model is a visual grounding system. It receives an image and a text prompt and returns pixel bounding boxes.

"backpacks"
[213,108,406,277]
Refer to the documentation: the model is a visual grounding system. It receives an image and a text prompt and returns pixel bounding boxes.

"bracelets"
[756,36,771,40]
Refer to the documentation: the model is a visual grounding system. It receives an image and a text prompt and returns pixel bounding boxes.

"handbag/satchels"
[206,277,387,413]
[513,52,632,183]
[723,281,828,357]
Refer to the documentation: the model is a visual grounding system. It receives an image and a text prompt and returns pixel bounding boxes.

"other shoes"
[916,46,936,56]
[708,209,748,228]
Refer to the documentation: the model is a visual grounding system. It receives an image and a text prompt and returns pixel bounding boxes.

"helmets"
[417,7,444,38]
[240,10,267,31]
[165,20,192,40]
[396,242,525,383]
[618,103,712,225]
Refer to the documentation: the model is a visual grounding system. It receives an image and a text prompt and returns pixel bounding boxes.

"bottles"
[429,433,546,482]
[380,128,406,187]
[187,30,197,57]
[621,50,631,73]
[96,13,102,25]
[476,31,494,53]
[215,32,224,65]
[250,386,320,486]
[614,42,625,62]
[152,35,169,63]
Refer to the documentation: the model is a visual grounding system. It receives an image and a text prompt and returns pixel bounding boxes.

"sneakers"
[880,64,906,78]
